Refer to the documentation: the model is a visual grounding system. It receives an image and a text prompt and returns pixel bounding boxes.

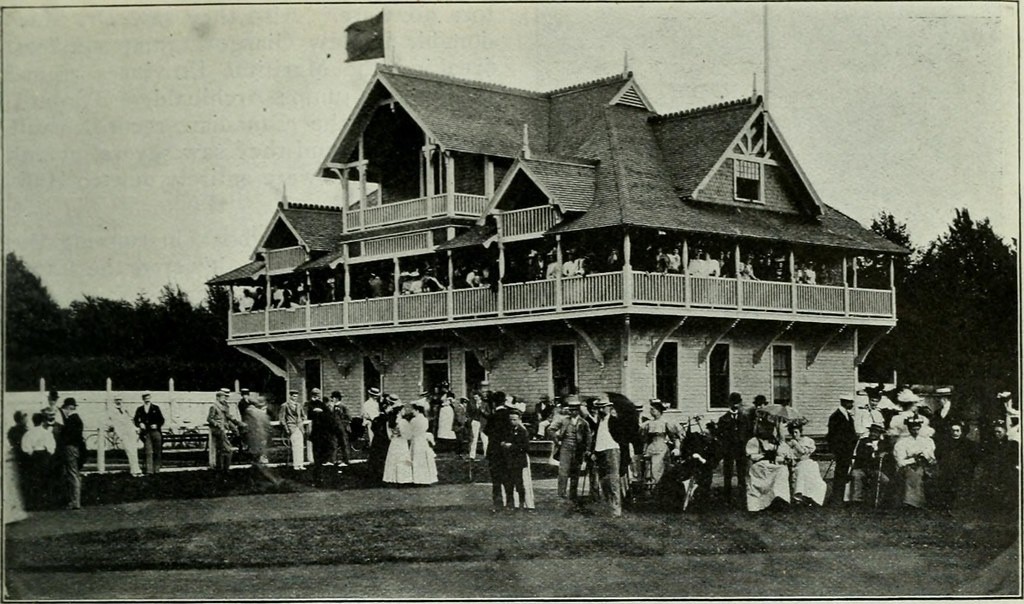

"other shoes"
[338,462,348,466]
[322,462,334,466]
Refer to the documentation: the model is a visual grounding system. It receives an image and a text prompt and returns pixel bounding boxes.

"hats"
[241,388,249,394]
[867,424,885,432]
[47,390,59,397]
[753,395,769,405]
[840,394,854,401]
[221,388,231,396]
[728,392,743,404]
[291,390,299,394]
[650,401,668,412]
[386,394,401,403]
[14,411,27,422]
[569,401,581,409]
[312,387,321,394]
[62,398,79,408]
[368,387,381,395]
[593,399,613,407]
[331,391,343,397]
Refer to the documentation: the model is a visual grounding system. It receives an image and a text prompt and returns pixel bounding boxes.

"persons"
[279,390,307,470]
[715,392,827,513]
[828,387,1018,512]
[206,388,275,480]
[362,380,456,487]
[656,247,736,277]
[530,238,620,280]
[239,280,307,313]
[7,388,83,510]
[535,394,622,518]
[307,388,351,467]
[108,395,144,477]
[680,421,722,492]
[639,402,680,489]
[400,258,493,296]
[453,390,534,511]
[740,251,833,285]
[133,393,164,477]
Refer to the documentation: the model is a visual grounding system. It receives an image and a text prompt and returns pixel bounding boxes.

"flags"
[346,10,383,62]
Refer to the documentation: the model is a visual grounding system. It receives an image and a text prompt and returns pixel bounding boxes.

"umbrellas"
[604,392,639,430]
[759,403,803,426]
